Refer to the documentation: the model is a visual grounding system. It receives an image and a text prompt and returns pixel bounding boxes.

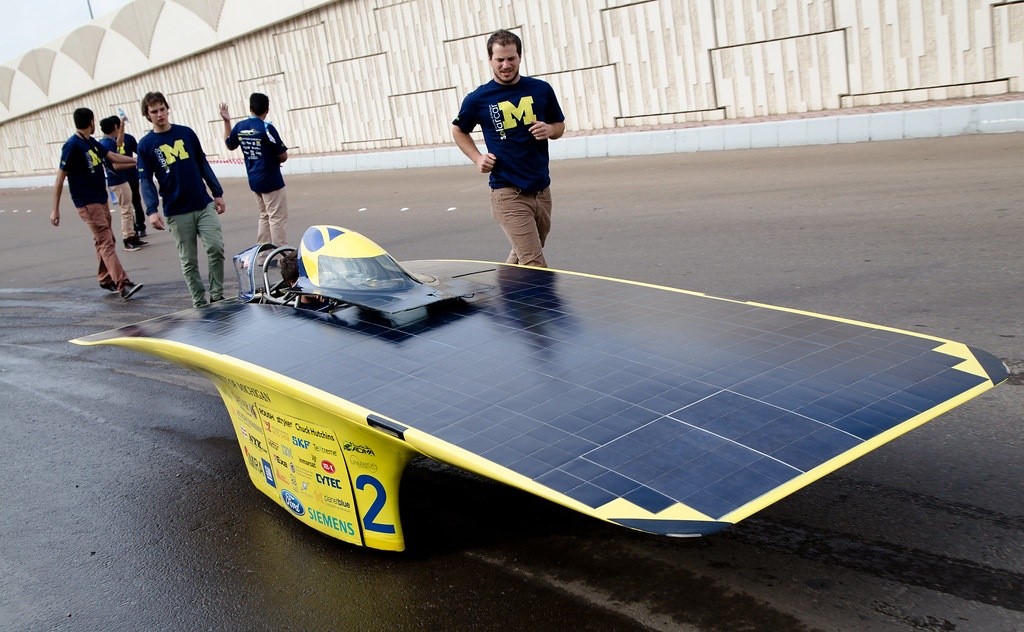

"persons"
[280,249,327,310]
[99,118,149,251]
[50,108,144,300]
[218,92,288,266]
[107,115,147,238]
[137,91,226,307]
[451,28,565,268]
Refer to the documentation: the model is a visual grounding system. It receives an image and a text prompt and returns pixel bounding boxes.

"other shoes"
[210,296,225,303]
[123,236,148,252]
[139,230,146,237]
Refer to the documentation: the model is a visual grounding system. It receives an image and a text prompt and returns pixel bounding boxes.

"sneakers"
[122,283,143,299]
[100,281,120,293]
